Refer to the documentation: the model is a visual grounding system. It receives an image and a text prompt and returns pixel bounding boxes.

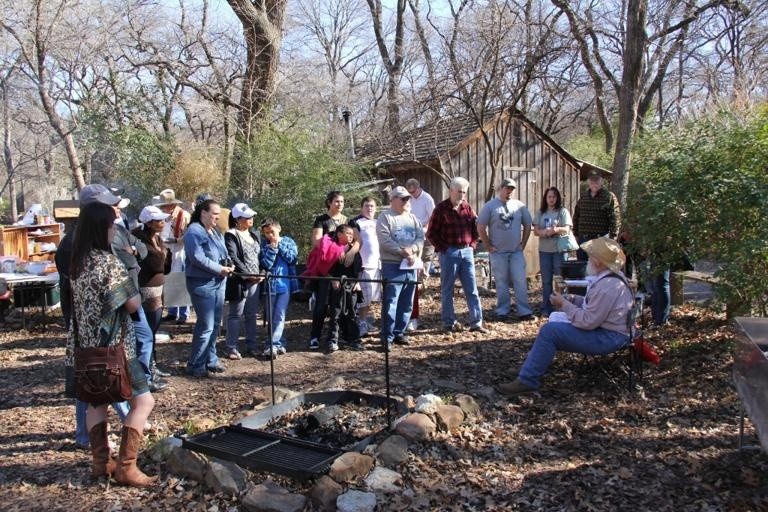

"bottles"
[544,216,551,229]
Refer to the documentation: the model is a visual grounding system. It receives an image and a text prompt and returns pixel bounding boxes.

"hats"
[138,205,171,224]
[579,236,627,275]
[391,186,412,200]
[195,193,213,207]
[231,202,258,219]
[151,188,183,207]
[106,186,131,209]
[79,183,122,207]
[501,178,517,189]
[587,168,602,179]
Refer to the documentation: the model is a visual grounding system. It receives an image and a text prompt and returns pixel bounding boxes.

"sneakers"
[444,321,467,332]
[468,325,490,334]
[496,378,539,396]
[176,316,190,325]
[277,341,287,355]
[262,345,278,360]
[357,320,368,338]
[308,335,322,350]
[382,338,393,353]
[220,346,243,360]
[185,361,209,378]
[349,341,365,352]
[507,366,522,376]
[394,334,412,346]
[496,314,507,322]
[206,358,226,373]
[146,373,166,383]
[367,322,378,332]
[520,312,538,320]
[147,380,168,393]
[327,338,340,351]
[150,366,172,378]
[160,314,177,321]
[244,345,262,358]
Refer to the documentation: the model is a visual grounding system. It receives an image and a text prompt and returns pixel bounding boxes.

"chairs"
[576,298,644,396]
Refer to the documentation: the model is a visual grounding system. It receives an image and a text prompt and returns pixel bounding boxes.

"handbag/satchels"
[70,343,133,406]
[553,230,580,255]
[223,275,246,304]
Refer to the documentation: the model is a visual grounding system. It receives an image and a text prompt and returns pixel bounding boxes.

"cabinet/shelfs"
[1,222,61,274]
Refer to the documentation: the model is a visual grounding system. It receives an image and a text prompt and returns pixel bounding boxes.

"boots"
[86,422,118,480]
[114,425,160,488]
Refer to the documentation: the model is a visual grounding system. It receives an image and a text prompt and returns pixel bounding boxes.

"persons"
[572,169,621,261]
[306,180,436,352]
[54,178,191,453]
[639,263,672,325]
[61,202,161,490]
[425,176,489,334]
[531,186,573,316]
[477,177,533,318]
[494,236,634,396]
[183,193,298,378]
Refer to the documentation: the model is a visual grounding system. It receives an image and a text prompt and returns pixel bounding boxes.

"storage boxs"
[13,282,60,306]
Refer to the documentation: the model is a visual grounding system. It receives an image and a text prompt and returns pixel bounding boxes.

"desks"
[1,273,59,332]
